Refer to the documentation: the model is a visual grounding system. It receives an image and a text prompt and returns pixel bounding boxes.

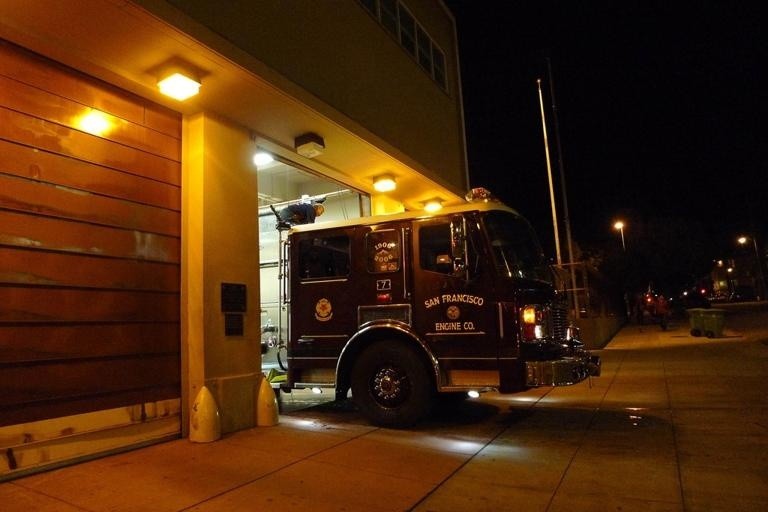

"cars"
[711,285,754,303]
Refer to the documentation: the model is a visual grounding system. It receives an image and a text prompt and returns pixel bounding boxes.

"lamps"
[373,175,399,194]
[153,62,203,100]
[295,131,327,157]
[420,196,445,212]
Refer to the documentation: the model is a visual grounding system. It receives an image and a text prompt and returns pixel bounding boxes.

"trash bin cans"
[687,310,726,337]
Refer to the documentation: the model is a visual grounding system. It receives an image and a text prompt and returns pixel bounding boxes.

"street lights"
[613,220,625,250]
[712,253,735,290]
[738,235,768,299]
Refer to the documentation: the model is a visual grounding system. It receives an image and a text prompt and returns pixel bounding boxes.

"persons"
[655,295,671,331]
[277,204,324,226]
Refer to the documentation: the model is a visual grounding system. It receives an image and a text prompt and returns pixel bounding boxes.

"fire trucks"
[259,187,601,428]
